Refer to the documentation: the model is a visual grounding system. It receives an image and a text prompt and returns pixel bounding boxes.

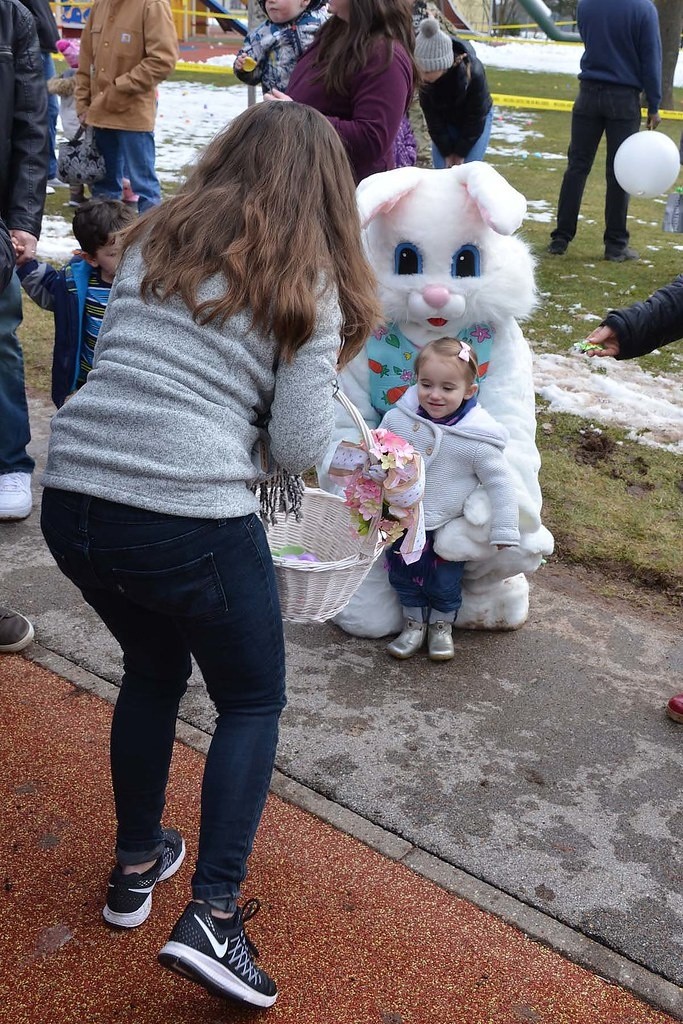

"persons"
[47,37,85,208]
[264,0,424,189]
[10,198,139,409]
[40,99,408,1012]
[392,113,417,168]
[121,87,158,211]
[232,0,334,96]
[547,1,662,262]
[579,271,683,361]
[75,0,179,214]
[414,17,494,170]
[374,336,520,659]
[0,0,61,520]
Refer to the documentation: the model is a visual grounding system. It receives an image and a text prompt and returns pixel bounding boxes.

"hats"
[413,18,454,70]
[58,37,81,69]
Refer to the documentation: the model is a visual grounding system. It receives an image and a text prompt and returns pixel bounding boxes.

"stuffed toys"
[312,160,554,639]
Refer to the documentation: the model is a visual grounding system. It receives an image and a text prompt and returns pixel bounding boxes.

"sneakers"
[605,247,639,262]
[102,830,184,927]
[0,471,32,519]
[0,608,35,653]
[158,901,279,1008]
[68,192,89,206]
[548,239,568,254]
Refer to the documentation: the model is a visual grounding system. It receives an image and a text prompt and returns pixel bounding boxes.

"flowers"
[342,427,415,544]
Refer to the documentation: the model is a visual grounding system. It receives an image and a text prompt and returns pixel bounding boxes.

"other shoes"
[46,186,56,194]
[388,620,428,658]
[428,621,455,660]
[668,694,683,722]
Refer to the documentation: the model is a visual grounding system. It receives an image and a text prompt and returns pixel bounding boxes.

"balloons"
[614,130,680,198]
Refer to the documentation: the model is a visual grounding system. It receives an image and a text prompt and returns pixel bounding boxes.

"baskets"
[256,387,383,626]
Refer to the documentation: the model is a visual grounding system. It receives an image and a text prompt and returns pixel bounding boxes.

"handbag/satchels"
[392,115,418,169]
[57,124,107,185]
[662,185,682,231]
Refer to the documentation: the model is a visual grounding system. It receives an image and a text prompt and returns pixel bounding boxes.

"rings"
[31,249,36,254]
